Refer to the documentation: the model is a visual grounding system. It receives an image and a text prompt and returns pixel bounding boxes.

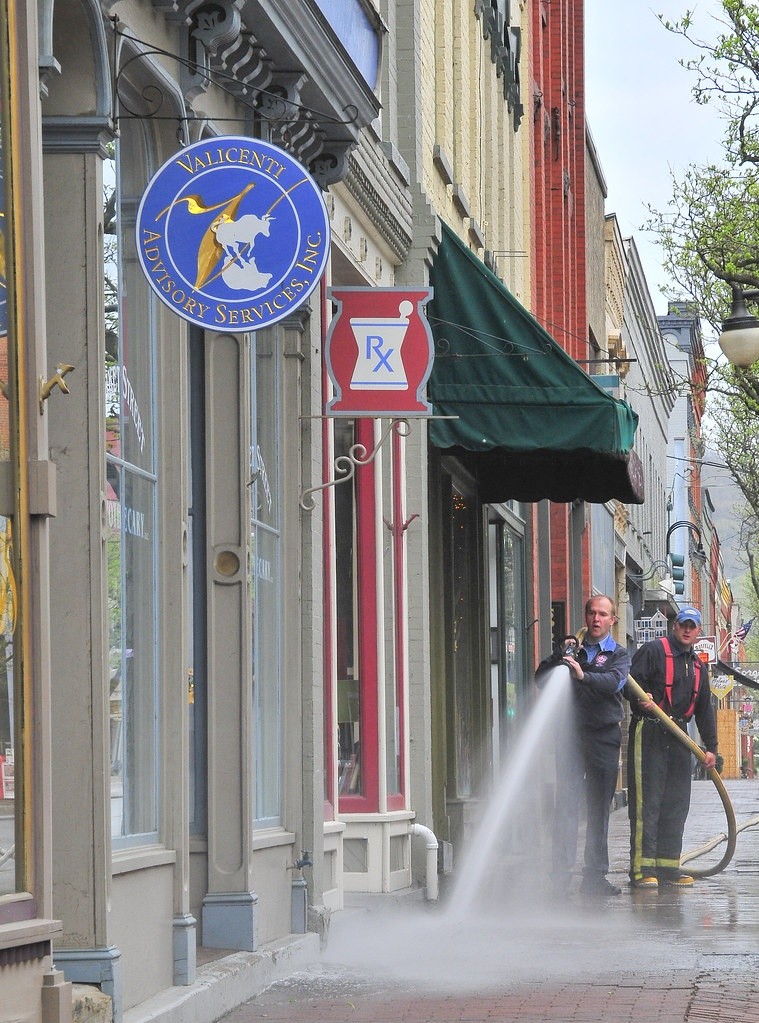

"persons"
[621,608,718,886]
[534,595,631,895]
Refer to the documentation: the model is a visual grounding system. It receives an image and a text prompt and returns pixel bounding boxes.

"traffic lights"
[668,552,685,595]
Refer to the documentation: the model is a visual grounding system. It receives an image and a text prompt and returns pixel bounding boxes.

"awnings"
[429,225,645,505]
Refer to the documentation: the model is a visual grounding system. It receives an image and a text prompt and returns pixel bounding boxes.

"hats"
[675,606,701,628]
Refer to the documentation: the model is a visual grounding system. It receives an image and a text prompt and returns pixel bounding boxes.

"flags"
[734,618,754,643]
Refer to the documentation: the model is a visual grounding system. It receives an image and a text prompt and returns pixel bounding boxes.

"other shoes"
[579,876,621,895]
[631,877,659,888]
[658,873,694,885]
